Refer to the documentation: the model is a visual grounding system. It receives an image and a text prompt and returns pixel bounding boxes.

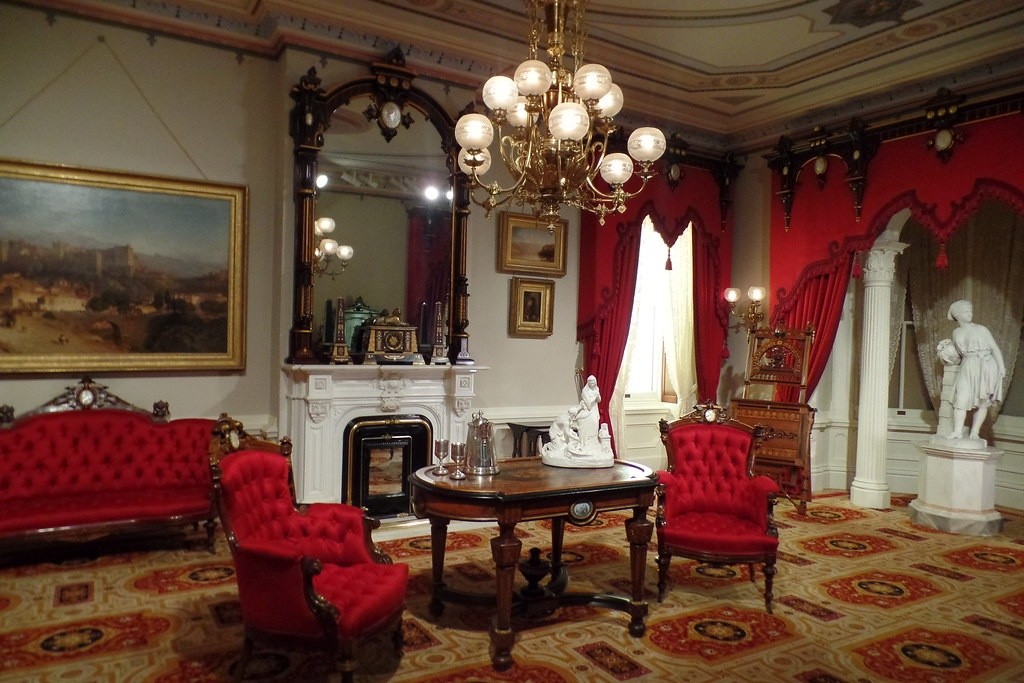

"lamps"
[313,216,354,280]
[725,285,766,322]
[454,0,666,234]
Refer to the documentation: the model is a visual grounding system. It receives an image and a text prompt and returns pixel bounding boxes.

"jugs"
[462,410,500,475]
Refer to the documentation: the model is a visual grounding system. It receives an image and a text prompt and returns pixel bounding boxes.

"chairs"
[208,411,410,683]
[656,399,781,615]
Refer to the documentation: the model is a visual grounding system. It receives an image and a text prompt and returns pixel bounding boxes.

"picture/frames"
[498,209,569,277]
[0,154,247,373]
[509,274,555,337]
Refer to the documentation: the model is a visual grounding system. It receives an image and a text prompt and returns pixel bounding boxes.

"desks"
[407,457,660,672]
[506,420,554,457]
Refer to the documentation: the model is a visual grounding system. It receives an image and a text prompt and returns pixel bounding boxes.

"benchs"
[0,375,269,552]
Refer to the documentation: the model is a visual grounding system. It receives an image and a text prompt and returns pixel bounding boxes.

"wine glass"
[450,443,466,480]
[432,439,450,475]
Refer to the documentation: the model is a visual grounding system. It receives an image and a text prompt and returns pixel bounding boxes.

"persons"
[936,299,1006,440]
[543,375,602,452]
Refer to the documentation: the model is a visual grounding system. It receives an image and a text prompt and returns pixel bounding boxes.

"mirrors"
[284,44,470,364]
